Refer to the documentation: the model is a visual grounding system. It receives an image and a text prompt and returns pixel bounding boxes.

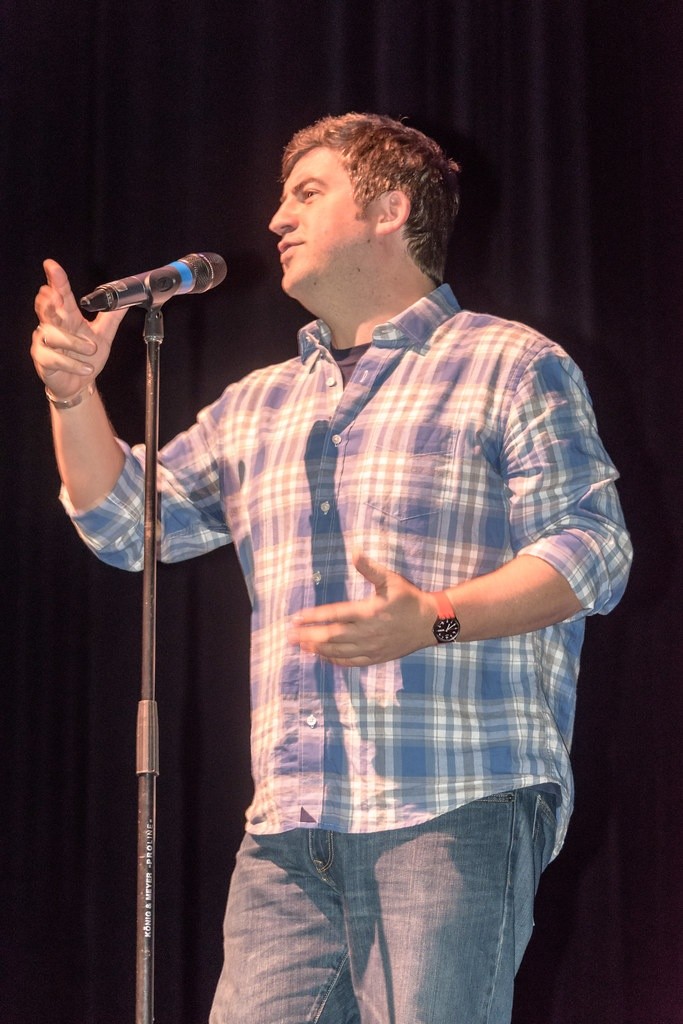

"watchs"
[429,588,460,645]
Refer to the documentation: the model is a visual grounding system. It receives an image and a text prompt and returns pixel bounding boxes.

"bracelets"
[44,382,95,410]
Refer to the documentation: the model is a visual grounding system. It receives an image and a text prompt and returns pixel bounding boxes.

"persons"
[29,114,633,1024]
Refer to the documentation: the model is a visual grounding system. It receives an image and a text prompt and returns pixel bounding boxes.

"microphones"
[79,252,228,313]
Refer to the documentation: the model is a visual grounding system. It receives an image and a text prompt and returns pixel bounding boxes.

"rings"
[36,326,49,345]
